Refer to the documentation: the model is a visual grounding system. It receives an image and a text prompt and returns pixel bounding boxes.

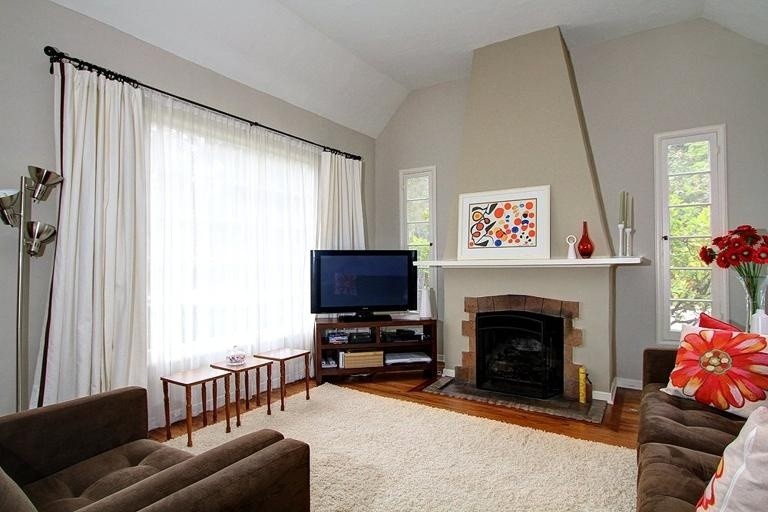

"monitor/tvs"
[309,249,417,322]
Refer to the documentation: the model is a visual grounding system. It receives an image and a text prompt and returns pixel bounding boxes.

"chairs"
[1,385,309,512]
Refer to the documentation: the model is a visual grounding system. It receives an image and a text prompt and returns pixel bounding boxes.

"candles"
[618,190,632,228]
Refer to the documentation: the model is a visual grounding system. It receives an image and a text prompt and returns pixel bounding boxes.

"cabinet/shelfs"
[313,314,437,386]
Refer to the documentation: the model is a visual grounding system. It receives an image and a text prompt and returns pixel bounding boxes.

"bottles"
[577,366,587,404]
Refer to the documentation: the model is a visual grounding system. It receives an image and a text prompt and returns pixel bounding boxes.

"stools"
[159,347,310,447]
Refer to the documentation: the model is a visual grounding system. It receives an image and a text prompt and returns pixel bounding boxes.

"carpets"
[160,382,638,511]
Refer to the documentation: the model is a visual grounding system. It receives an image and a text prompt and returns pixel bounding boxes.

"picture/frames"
[457,185,550,260]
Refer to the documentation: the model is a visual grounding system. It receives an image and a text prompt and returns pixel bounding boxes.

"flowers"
[698,225,768,314]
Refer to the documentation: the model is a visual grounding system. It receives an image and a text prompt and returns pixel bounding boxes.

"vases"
[737,276,767,332]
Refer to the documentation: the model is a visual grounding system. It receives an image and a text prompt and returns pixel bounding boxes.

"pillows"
[660,312,768,511]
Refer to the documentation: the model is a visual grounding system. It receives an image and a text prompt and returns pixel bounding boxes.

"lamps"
[0,164,65,415]
